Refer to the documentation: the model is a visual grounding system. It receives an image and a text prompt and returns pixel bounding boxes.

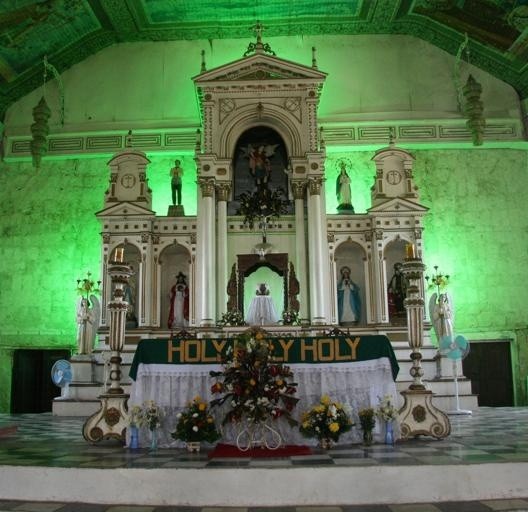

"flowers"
[300,394,398,440]
[208,329,302,423]
[126,394,217,443]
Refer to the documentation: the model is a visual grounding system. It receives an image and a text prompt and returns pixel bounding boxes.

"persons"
[167,272,189,326]
[433,294,456,347]
[121,266,136,320]
[170,160,184,206]
[339,271,360,324]
[76,299,96,357]
[390,263,406,306]
[248,144,271,191]
[337,166,351,206]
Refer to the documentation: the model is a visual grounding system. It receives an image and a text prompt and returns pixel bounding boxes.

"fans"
[437,332,473,415]
[49,359,77,399]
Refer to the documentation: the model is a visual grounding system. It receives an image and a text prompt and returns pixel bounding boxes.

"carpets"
[207,440,311,458]
